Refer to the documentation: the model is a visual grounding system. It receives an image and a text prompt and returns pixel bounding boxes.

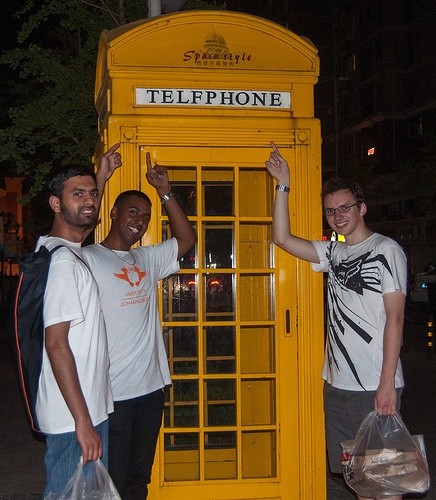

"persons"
[265,141,407,500]
[13,143,197,500]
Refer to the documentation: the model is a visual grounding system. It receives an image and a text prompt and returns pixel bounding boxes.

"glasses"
[322,203,357,216]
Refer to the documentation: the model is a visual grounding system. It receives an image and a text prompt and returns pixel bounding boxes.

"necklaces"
[101,241,136,266]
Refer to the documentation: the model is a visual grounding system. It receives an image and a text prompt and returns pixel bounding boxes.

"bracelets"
[160,191,173,205]
[275,184,290,194]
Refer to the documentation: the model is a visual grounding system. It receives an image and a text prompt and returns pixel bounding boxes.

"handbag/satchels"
[58,456,121,500]
[340,411,430,498]
[11,245,94,439]
